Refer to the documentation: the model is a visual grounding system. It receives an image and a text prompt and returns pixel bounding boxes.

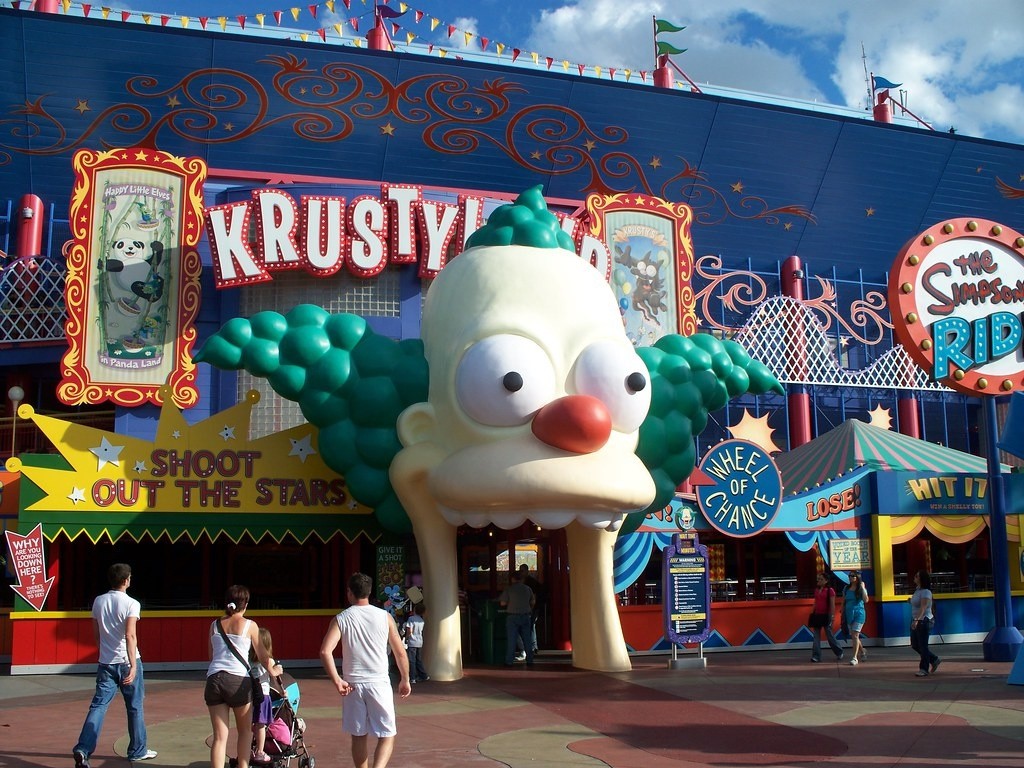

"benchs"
[723,593,752,596]
[628,576,798,602]
[760,591,797,595]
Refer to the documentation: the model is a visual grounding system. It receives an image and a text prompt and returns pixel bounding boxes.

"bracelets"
[913,618,919,621]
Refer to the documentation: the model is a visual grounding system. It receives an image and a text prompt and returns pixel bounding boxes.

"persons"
[840,570,869,665]
[319,573,412,768]
[810,573,845,662]
[910,571,942,676]
[73,563,158,768]
[204,586,283,768]
[513,563,540,660]
[246,627,289,761]
[498,570,535,667]
[406,603,430,684]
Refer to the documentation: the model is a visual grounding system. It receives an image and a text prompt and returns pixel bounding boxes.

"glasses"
[848,574,857,577]
[915,575,919,578]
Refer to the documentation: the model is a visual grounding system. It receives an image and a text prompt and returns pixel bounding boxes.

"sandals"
[255,751,270,760]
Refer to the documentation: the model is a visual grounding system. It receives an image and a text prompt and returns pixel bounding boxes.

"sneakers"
[130,747,158,762]
[74,752,90,768]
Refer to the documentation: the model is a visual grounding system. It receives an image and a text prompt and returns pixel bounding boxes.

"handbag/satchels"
[809,613,830,627]
[251,679,265,705]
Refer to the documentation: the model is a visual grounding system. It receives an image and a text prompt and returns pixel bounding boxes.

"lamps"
[7,385,24,412]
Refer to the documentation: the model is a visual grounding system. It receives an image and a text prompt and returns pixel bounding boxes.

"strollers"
[229,659,317,768]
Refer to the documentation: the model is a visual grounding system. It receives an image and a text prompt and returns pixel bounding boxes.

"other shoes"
[527,663,532,665]
[931,658,941,673]
[850,658,859,665]
[811,657,818,661]
[423,676,430,681]
[861,652,867,662]
[838,651,843,659]
[515,651,526,661]
[410,681,417,684]
[916,671,928,676]
[503,662,512,667]
